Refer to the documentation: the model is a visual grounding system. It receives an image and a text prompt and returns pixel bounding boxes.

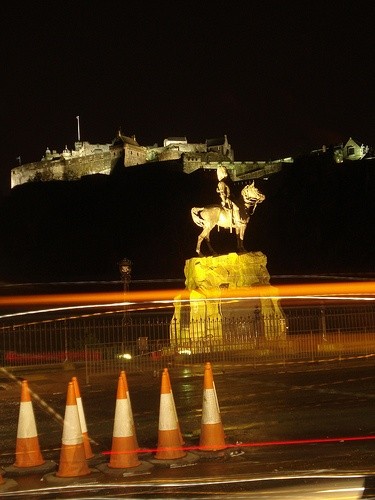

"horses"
[191,181,265,257]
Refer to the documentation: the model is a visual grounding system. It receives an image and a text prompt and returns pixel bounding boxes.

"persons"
[217,160,235,213]
